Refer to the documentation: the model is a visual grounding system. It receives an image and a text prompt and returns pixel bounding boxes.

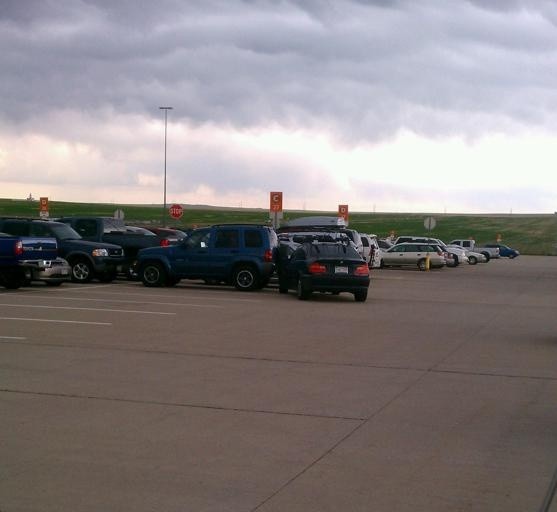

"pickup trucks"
[449,239,500,261]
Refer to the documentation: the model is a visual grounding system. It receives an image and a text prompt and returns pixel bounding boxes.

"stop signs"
[169,204,183,220]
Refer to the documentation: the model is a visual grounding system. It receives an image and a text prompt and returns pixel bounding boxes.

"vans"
[381,237,485,271]
[277,217,394,301]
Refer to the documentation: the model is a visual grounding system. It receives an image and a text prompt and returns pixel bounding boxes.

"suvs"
[0,214,189,289]
[136,224,278,292]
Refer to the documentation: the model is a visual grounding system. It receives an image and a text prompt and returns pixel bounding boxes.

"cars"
[486,244,520,259]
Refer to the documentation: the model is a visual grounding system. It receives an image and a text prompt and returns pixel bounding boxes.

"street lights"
[159,105,168,222]
